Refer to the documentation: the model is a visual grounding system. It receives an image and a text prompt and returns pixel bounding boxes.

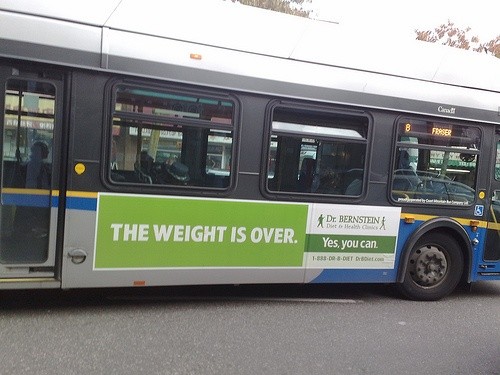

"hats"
[167,163,192,183]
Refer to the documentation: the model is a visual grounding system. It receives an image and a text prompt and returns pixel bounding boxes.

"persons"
[19,141,53,238]
[397,150,424,190]
[140,150,165,184]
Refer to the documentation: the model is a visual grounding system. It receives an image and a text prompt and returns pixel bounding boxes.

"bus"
[0,2,500,303]
